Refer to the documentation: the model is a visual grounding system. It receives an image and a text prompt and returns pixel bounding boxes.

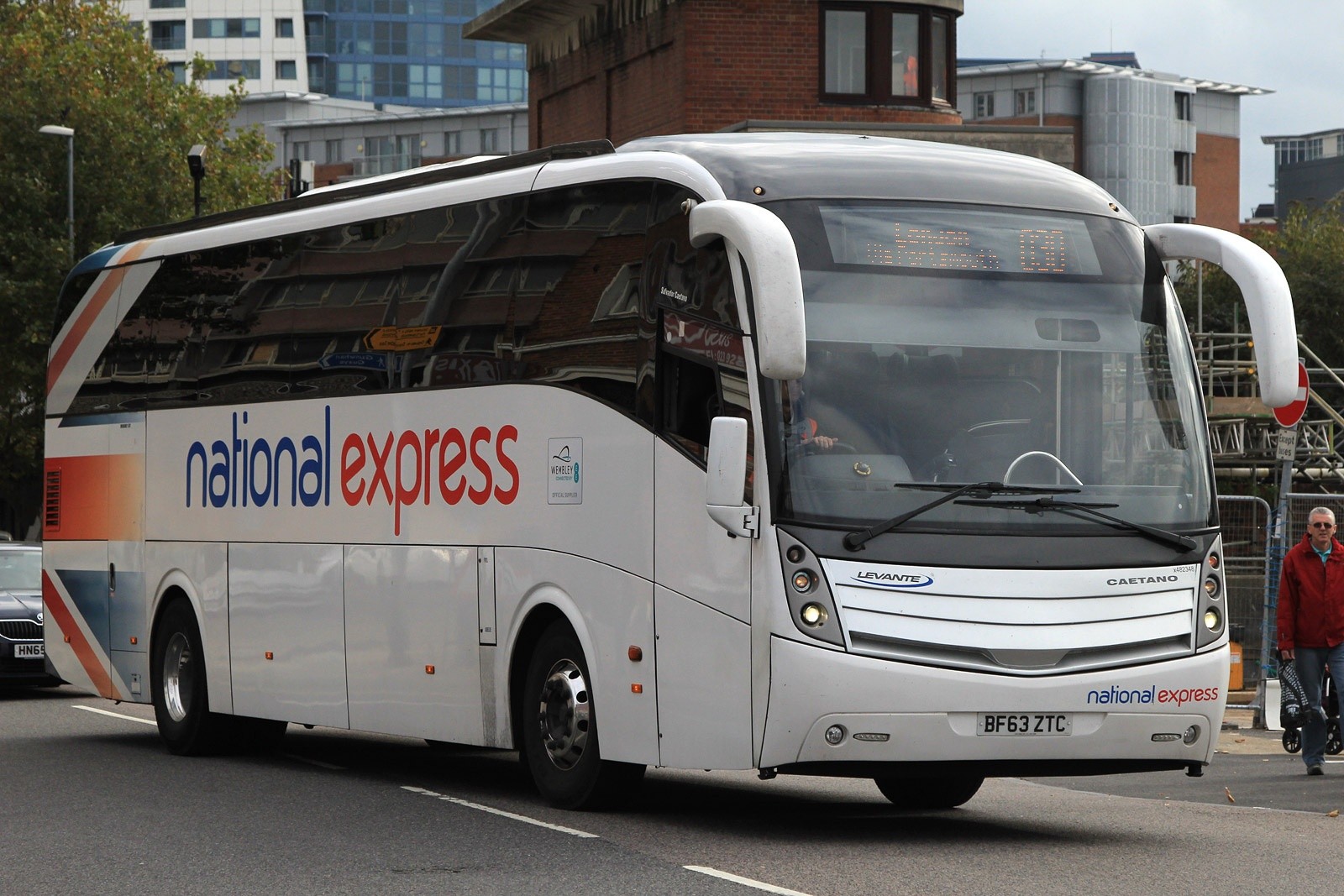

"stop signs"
[1271,361,1309,427]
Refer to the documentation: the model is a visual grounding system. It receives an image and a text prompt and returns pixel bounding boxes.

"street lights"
[38,123,76,267]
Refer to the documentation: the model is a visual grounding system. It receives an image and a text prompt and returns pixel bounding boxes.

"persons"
[775,380,839,449]
[1277,507,1344,776]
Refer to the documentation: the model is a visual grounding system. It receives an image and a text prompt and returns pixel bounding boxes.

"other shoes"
[1306,763,1325,775]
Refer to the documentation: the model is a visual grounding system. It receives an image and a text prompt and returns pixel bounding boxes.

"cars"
[0,532,70,689]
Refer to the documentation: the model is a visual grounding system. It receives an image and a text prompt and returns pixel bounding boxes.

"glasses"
[1308,522,1334,529]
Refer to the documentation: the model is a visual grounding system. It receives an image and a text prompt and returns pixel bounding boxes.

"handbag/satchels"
[1278,657,1311,732]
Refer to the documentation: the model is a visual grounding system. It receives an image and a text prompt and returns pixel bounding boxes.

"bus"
[41,131,1312,817]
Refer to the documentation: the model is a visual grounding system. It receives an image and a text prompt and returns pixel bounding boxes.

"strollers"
[1275,647,1344,756]
